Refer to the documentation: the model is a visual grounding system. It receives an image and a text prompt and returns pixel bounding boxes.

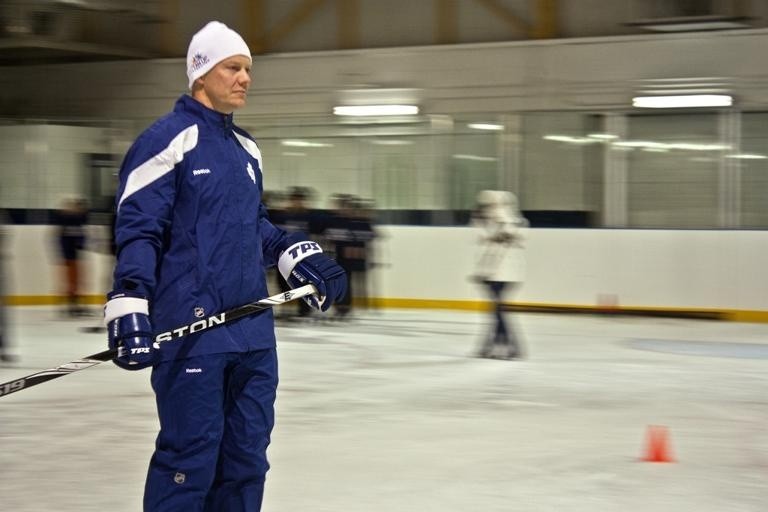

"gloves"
[278,232,347,311]
[103,290,156,370]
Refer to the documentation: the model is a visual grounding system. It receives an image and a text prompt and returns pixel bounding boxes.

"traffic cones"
[640,421,677,463]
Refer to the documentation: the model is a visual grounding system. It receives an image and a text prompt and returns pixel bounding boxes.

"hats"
[187,21,252,90]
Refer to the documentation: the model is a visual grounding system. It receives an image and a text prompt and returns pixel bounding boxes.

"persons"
[59,197,92,316]
[465,189,528,359]
[254,189,379,320]
[102,21,348,512]
